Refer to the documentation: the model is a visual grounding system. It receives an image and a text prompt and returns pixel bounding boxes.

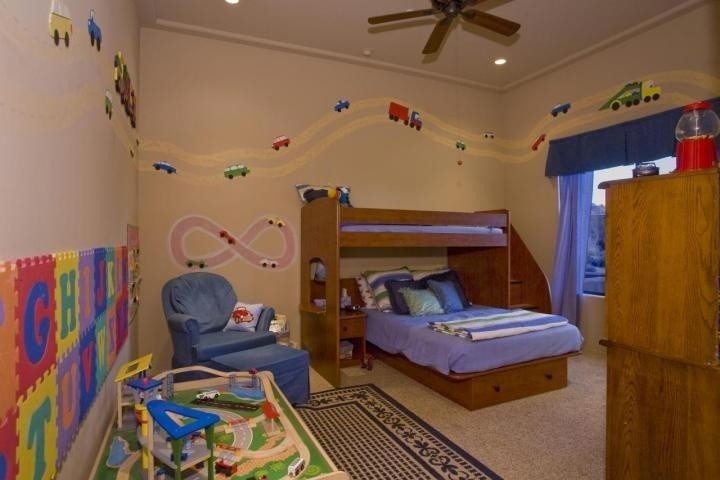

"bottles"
[674,101,720,173]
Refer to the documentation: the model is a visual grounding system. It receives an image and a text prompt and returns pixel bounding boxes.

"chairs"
[161,272,276,369]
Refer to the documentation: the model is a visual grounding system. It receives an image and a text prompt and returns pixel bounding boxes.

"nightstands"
[340,310,368,369]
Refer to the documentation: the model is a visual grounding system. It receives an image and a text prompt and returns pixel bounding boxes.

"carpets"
[292,383,505,480]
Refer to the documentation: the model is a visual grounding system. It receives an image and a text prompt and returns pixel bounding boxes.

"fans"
[368,0,521,54]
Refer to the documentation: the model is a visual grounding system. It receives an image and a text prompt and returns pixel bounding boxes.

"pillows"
[355,264,470,317]
[170,272,237,335]
[223,302,265,333]
[295,184,353,208]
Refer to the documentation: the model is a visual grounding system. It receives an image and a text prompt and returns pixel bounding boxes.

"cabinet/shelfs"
[598,168,720,480]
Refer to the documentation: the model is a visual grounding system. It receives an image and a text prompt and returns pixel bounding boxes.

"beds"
[299,197,552,389]
[340,267,583,411]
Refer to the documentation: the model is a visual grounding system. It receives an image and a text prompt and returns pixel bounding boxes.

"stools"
[211,343,310,406]
